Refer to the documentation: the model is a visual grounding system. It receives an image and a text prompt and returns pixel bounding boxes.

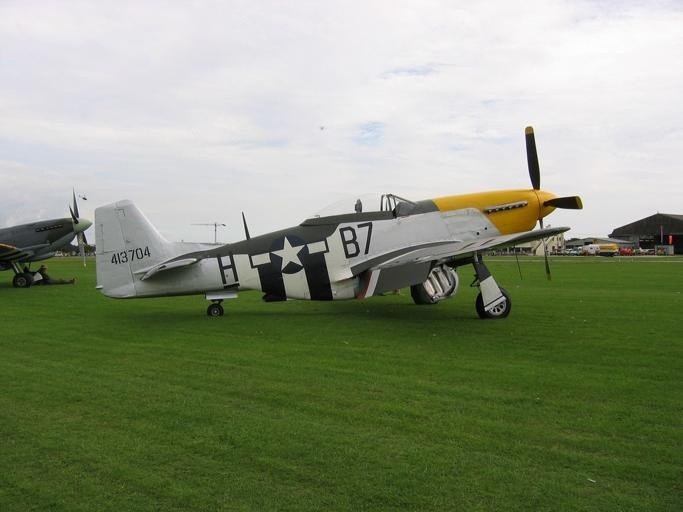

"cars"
[564,244,654,255]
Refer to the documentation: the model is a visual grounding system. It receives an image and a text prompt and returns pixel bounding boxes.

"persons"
[36,265,77,285]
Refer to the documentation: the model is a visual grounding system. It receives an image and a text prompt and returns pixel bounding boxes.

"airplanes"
[95,124,584,318]
[0,189,93,289]
